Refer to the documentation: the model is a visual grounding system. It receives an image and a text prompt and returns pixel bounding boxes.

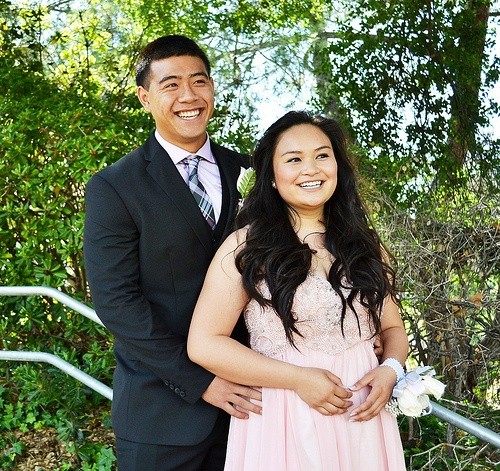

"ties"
[183,156,216,230]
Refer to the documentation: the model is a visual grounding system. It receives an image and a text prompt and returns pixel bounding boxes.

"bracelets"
[379,358,447,419]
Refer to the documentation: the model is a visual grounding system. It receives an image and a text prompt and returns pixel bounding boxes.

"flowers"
[234,166,258,221]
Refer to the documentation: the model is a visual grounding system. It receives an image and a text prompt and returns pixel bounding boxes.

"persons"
[187,110,409,471]
[83,35,258,471]
